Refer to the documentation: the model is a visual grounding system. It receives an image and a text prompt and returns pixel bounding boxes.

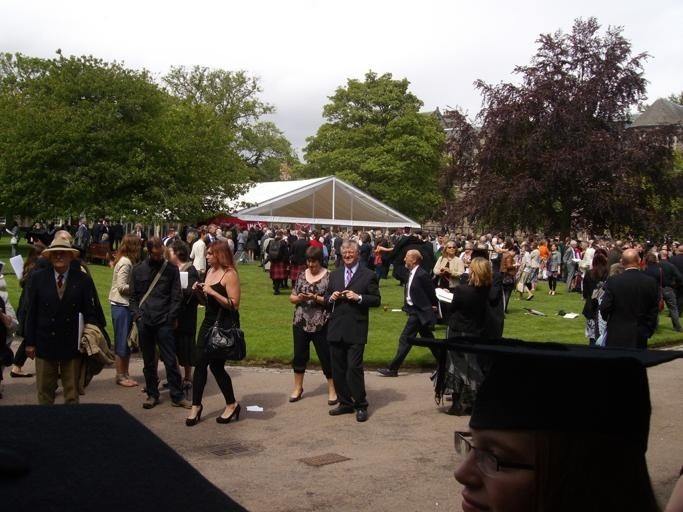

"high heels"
[186,404,202,426]
[216,403,240,423]
[328,396,338,405]
[290,388,303,402]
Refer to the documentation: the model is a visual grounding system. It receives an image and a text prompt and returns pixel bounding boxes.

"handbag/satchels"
[128,321,139,353]
[196,327,246,360]
[503,275,516,288]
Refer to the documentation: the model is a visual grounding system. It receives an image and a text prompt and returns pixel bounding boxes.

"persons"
[405,331,683,510]
[1,213,683,433]
[664,460,683,512]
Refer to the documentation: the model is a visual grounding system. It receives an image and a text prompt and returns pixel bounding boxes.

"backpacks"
[268,240,282,261]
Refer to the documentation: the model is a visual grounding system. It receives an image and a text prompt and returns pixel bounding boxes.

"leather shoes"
[376,368,397,377]
[357,408,367,422]
[10,371,33,378]
[329,406,353,415]
[183,377,192,390]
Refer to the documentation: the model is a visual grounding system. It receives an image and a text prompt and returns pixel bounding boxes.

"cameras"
[196,283,204,292]
[339,293,346,297]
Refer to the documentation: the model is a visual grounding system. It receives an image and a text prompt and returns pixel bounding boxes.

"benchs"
[86,244,108,265]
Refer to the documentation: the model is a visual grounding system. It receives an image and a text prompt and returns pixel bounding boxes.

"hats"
[42,237,80,260]
[401,333,683,441]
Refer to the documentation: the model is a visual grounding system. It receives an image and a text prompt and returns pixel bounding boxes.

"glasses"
[447,246,456,249]
[454,431,536,479]
[341,251,357,255]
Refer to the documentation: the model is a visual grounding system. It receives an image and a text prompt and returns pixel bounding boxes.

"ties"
[345,270,353,289]
[57,274,64,288]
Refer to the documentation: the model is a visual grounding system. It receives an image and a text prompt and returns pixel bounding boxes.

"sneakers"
[116,374,139,387]
[171,395,192,409]
[143,396,161,409]
